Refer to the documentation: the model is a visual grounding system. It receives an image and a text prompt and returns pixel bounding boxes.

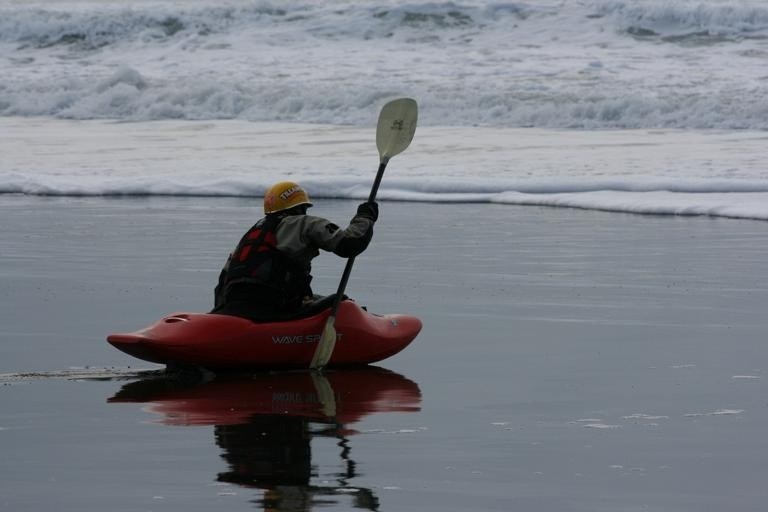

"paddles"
[309,98,418,368]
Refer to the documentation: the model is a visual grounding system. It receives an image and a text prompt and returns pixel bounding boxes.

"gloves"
[357,202,379,222]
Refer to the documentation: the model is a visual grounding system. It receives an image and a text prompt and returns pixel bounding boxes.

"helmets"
[264,183,313,216]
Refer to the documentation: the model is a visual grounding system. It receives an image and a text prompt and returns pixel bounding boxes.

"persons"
[214,181,378,323]
[214,415,359,511]
